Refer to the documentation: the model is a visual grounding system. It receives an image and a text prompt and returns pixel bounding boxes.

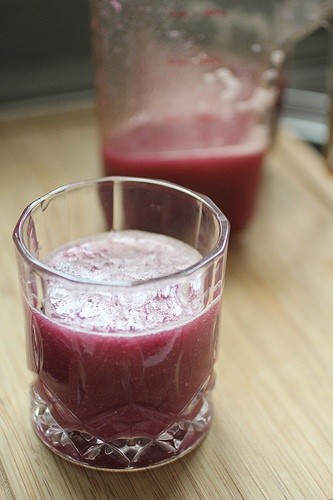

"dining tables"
[0,97,333,499]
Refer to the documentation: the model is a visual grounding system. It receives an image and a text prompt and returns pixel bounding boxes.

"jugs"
[89,0,330,242]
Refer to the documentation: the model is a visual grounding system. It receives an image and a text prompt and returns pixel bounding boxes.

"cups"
[14,175,231,472]
